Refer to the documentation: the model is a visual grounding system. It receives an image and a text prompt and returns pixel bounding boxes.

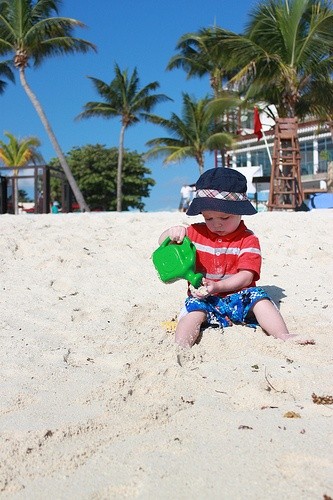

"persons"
[158,167,316,349]
[180,183,193,211]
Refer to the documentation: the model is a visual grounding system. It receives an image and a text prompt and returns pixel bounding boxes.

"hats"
[186,167,258,216]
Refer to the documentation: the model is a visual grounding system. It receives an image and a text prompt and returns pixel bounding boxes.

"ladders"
[266,116,303,212]
